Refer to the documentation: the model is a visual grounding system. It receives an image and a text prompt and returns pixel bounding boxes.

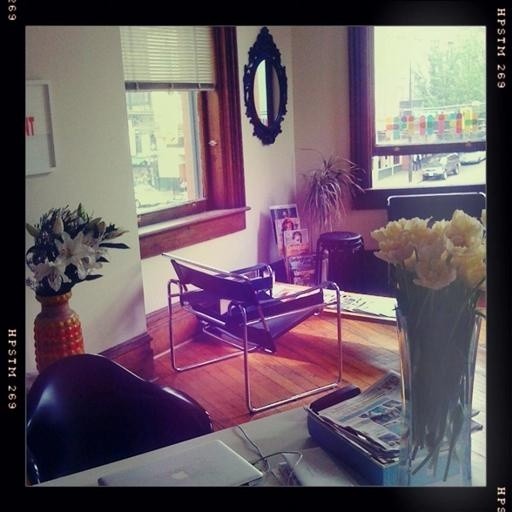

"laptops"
[96,439,264,487]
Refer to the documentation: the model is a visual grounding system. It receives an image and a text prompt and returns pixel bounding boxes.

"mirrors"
[243,27,288,145]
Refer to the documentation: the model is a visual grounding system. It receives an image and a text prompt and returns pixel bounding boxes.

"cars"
[460,150,486,163]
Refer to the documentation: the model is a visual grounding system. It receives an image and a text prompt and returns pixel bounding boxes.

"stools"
[316,232,367,289]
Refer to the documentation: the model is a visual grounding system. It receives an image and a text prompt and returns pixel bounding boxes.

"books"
[270,203,317,287]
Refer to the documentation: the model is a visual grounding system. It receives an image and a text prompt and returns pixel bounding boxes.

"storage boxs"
[308,400,461,484]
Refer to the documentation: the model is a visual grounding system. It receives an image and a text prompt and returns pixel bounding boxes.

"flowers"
[371,209,486,481]
[25,202,131,297]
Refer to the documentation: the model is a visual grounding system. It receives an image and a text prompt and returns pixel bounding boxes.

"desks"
[30,407,485,487]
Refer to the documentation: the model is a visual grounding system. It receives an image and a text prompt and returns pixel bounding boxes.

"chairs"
[162,252,342,413]
[26,354,214,484]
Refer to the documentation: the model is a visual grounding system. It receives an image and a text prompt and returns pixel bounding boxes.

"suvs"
[422,151,460,177]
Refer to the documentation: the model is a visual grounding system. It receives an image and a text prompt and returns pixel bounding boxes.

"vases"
[397,308,482,487]
[34,291,85,373]
